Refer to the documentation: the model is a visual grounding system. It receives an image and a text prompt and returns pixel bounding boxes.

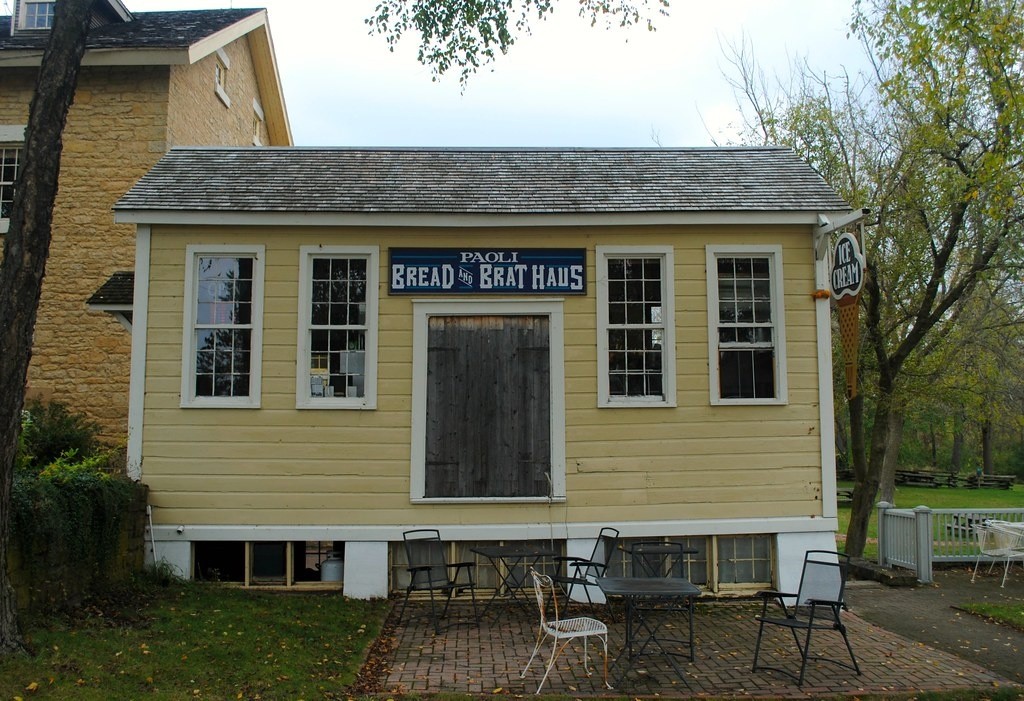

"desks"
[1004,522,1024,531]
[469,545,558,629]
[595,577,702,688]
[618,544,699,622]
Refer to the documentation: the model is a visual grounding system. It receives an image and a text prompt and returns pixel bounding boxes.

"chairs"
[396,529,480,636]
[751,550,862,686]
[969,523,1024,589]
[627,541,694,663]
[545,527,620,624]
[984,520,1024,574]
[519,566,613,696]
